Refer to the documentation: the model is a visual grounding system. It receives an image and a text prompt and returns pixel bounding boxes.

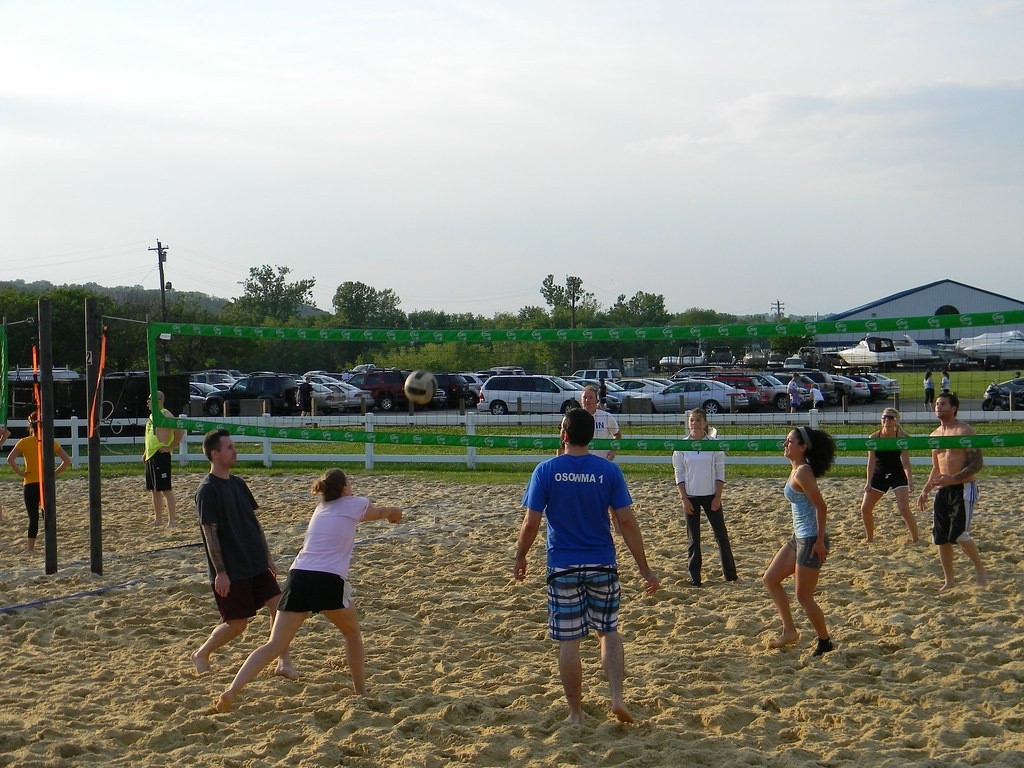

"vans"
[476,375,607,415]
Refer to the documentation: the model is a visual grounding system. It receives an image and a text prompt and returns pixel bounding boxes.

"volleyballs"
[403,369,439,405]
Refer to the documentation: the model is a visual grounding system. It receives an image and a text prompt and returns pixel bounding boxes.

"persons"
[6,411,71,552]
[762,426,836,659]
[0,427,11,449]
[922,368,952,409]
[786,371,826,414]
[215,468,403,711]
[673,407,744,587]
[861,407,923,548]
[189,429,306,681]
[1013,369,1022,379]
[142,390,186,529]
[513,377,661,724]
[917,392,985,593]
[298,374,314,416]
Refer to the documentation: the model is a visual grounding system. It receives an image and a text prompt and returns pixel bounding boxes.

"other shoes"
[813,637,833,657]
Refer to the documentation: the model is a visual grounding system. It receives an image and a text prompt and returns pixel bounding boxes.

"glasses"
[147,397,151,401]
[557,423,568,434]
[882,415,896,420]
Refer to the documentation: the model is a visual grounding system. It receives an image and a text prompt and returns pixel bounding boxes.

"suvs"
[707,376,768,412]
[757,375,811,410]
[201,373,298,416]
[346,369,412,412]
[572,370,622,382]
[670,366,724,378]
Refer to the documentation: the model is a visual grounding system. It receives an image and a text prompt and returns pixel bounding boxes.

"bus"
[985,377,1024,411]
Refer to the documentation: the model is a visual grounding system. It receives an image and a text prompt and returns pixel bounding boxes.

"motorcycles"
[982,380,1019,411]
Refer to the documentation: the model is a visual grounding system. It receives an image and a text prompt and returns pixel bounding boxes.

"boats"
[659,333,940,369]
[937,330,1024,361]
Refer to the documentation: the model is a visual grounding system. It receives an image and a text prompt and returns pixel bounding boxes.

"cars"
[291,371,343,383]
[634,380,748,414]
[295,380,375,414]
[7,370,153,382]
[783,371,900,406]
[949,357,968,371]
[353,364,377,371]
[558,376,683,412]
[432,366,526,409]
[188,370,249,415]
